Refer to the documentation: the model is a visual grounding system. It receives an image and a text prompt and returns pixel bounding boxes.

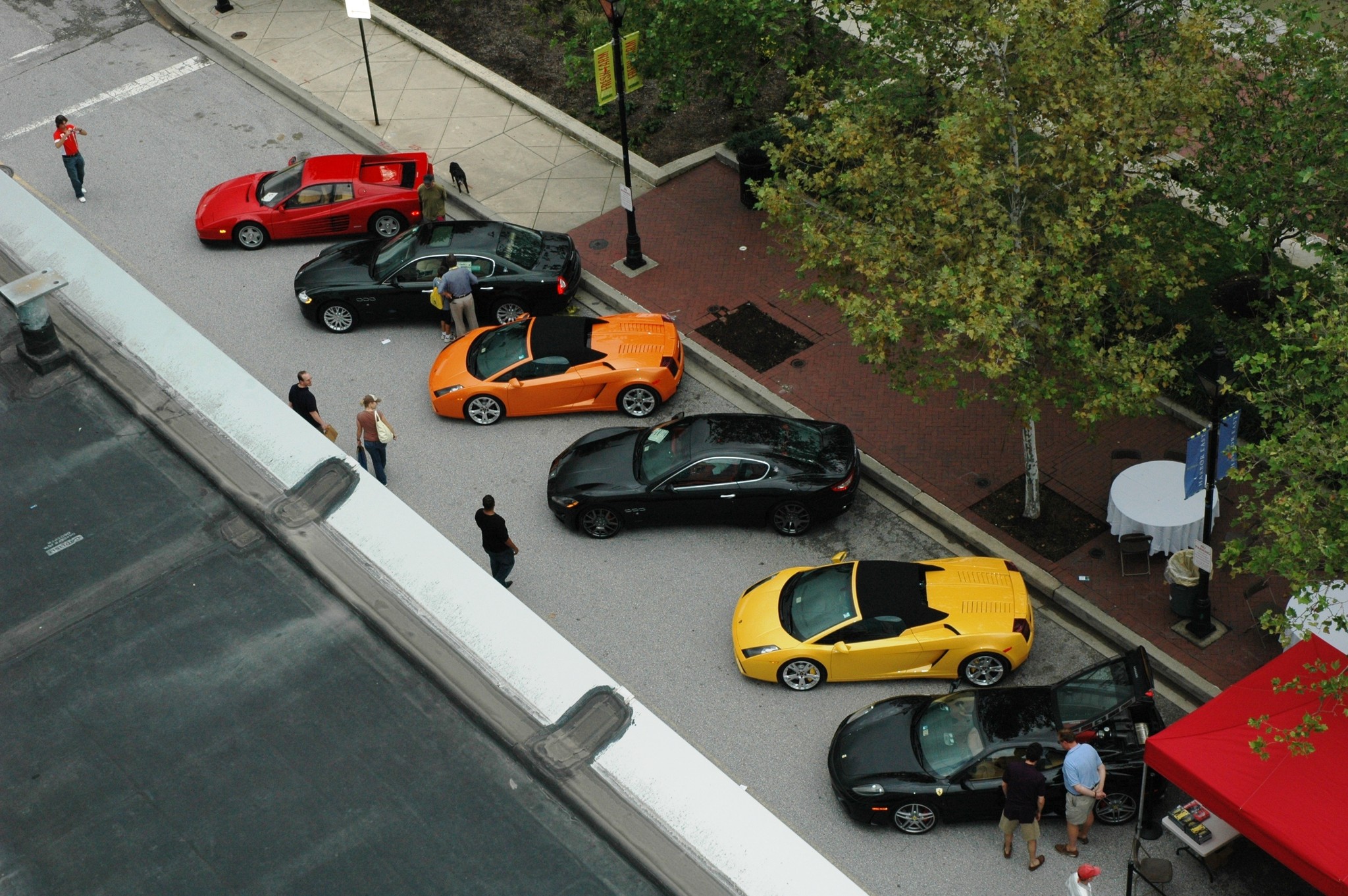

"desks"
[1162,799,1237,883]
[1106,460,1222,559]
[1279,581,1348,655]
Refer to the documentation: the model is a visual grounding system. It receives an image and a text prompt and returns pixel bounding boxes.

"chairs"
[417,258,445,281]
[299,186,331,204]
[1120,534,1153,577]
[1126,839,1173,896]
[1110,448,1143,482]
[1164,450,1190,463]
[685,454,744,487]
[974,750,1015,778]
[1046,751,1067,767]
[1244,577,1278,648]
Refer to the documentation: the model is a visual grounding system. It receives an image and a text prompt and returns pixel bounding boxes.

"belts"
[453,292,470,298]
[62,152,78,157]
[1068,783,1099,797]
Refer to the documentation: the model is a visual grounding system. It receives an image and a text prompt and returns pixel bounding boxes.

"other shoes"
[506,581,513,588]
[1003,841,1012,858]
[79,197,85,202]
[383,483,388,486]
[81,188,87,193]
[1029,855,1045,871]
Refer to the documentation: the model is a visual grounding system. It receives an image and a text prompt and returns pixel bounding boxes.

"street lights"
[597,0,649,271]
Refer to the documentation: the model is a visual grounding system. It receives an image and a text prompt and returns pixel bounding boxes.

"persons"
[288,371,327,434]
[1055,729,1106,858]
[433,255,478,343]
[475,494,518,589]
[1065,863,1100,896]
[356,394,397,487]
[999,743,1046,871]
[53,115,87,202]
[417,174,447,224]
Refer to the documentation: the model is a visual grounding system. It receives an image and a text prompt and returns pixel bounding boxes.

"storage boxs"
[1168,801,1215,845]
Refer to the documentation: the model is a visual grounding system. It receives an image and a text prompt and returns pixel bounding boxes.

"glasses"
[1058,740,1062,744]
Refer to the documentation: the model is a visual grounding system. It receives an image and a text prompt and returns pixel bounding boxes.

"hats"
[1078,864,1101,880]
[364,394,381,402]
[424,174,433,184]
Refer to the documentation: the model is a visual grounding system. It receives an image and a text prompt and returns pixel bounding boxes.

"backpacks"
[430,278,445,310]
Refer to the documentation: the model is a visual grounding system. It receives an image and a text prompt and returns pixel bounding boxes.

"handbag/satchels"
[325,424,338,442]
[374,410,393,444]
[357,446,368,471]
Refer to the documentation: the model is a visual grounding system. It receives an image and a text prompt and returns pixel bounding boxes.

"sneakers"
[445,335,457,342]
[1077,835,1088,844]
[441,332,455,340]
[1054,844,1079,858]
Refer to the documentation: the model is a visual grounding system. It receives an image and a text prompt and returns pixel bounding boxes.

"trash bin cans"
[1166,551,1214,617]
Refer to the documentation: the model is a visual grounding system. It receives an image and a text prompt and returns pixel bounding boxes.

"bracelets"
[321,423,325,428]
[79,129,82,132]
[357,439,360,441]
[1094,792,1096,798]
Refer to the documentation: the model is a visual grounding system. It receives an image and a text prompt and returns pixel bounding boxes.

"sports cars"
[546,411,863,538]
[731,551,1039,691]
[194,151,435,251]
[429,313,684,426]
[294,218,584,334]
[826,645,1172,835]
